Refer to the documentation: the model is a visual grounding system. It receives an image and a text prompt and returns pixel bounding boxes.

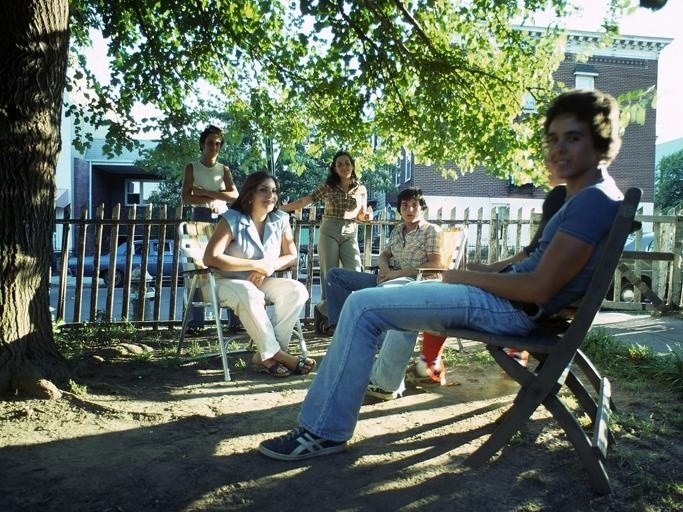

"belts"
[500,265,547,326]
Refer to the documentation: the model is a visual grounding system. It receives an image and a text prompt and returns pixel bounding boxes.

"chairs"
[413,226,468,352]
[464,186,645,497]
[174,218,307,384]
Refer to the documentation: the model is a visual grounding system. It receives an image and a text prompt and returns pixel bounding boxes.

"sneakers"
[184,326,202,337]
[259,427,349,462]
[366,379,398,400]
[313,305,328,335]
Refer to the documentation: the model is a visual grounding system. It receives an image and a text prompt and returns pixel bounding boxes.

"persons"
[276,151,374,337]
[258,86,626,463]
[324,185,447,349]
[180,123,245,338]
[199,169,318,378]
[405,143,568,385]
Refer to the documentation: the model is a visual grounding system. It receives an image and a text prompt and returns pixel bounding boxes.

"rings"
[256,277,261,281]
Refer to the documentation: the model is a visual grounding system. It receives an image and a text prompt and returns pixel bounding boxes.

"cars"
[66,238,183,288]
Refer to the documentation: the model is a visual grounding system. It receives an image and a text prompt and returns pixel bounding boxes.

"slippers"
[248,356,292,377]
[294,355,316,375]
